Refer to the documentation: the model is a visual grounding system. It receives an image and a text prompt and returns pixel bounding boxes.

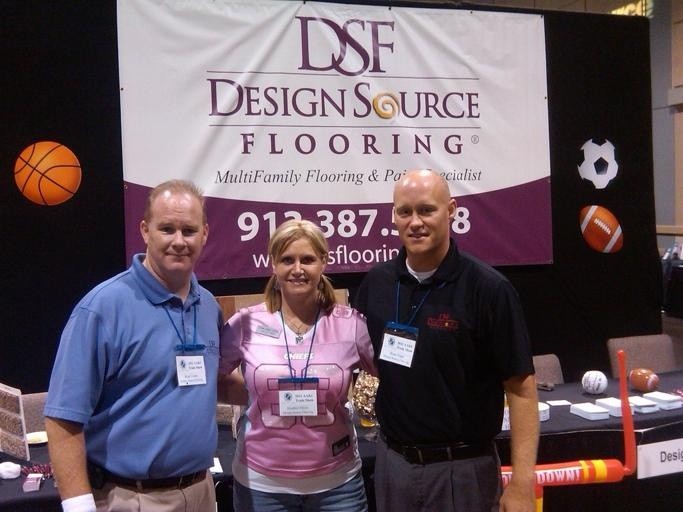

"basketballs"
[15,142,82,206]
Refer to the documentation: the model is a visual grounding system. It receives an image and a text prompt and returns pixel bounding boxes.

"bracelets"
[56,490,100,512]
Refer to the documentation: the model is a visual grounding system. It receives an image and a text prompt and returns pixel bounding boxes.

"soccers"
[577,137,622,189]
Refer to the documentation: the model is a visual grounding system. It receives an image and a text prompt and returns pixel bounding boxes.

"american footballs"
[630,368,659,392]
[581,206,623,253]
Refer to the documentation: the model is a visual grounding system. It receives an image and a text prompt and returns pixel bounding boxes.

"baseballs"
[582,371,607,394]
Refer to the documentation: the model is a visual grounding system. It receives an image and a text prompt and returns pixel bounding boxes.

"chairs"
[16,389,50,437]
[602,334,677,378]
[529,353,567,385]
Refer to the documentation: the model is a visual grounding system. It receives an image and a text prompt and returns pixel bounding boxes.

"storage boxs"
[211,285,350,406]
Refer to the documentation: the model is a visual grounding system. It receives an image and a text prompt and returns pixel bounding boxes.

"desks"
[1,367,682,512]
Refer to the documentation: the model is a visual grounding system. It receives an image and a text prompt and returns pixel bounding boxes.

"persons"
[41,179,245,512]
[218,218,379,510]
[353,169,541,510]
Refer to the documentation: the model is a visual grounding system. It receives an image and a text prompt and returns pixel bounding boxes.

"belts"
[386,439,489,465]
[86,464,207,489]
